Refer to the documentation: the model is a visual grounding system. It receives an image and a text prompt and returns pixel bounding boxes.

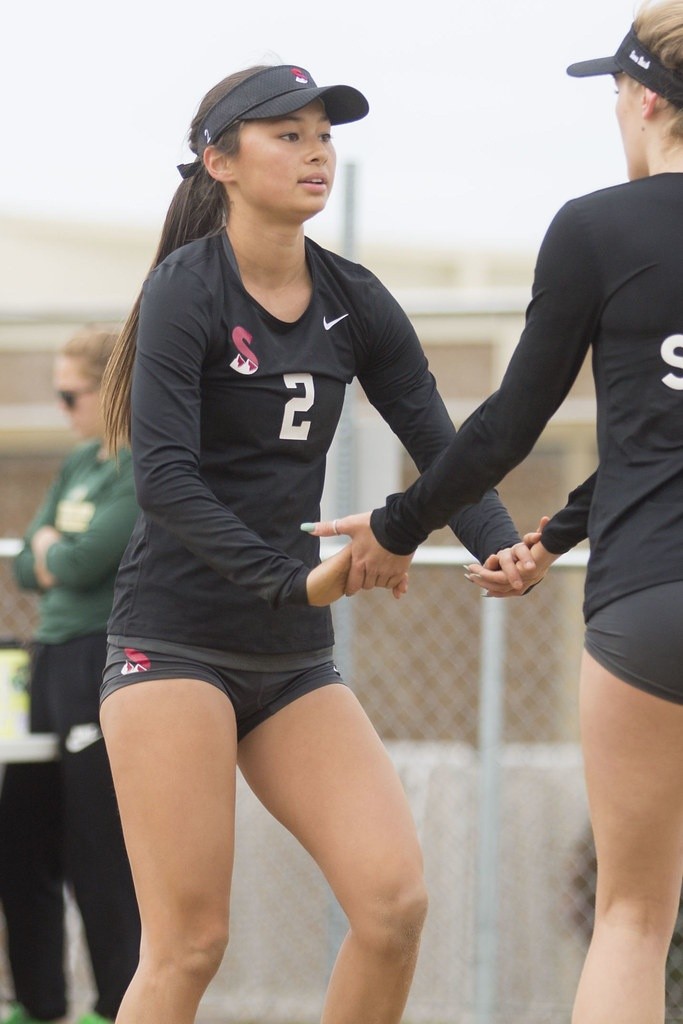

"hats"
[175,64,369,180]
[565,19,683,111]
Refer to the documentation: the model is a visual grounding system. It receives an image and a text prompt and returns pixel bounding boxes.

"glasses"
[57,379,107,411]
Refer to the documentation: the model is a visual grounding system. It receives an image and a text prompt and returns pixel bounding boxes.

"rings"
[332,520,341,537]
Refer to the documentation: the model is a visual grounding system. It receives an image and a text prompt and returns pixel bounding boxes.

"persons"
[296,0,683,1024]
[94,67,552,1024]
[4,322,159,1024]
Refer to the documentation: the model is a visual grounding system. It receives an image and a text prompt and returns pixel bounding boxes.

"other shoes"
[3,1003,64,1023]
[76,1009,114,1023]
